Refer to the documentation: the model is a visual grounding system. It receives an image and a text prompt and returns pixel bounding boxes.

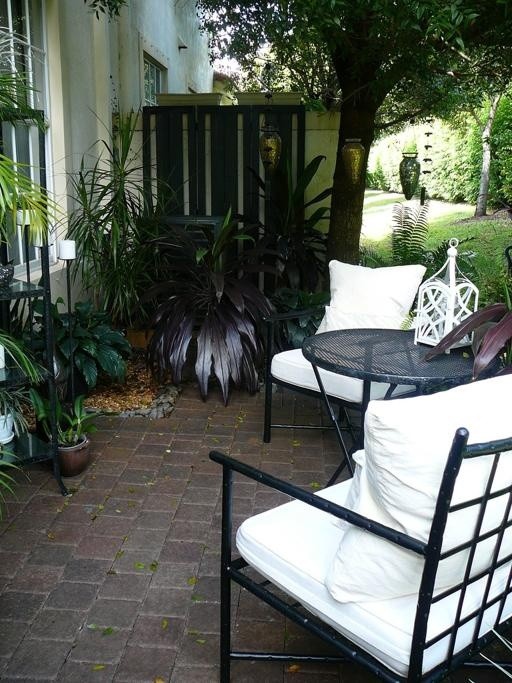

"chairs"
[205,422,512,682]
[258,290,417,454]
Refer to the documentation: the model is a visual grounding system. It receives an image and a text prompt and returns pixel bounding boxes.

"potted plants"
[0,149,135,476]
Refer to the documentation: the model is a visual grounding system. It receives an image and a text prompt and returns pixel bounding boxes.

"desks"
[300,327,503,501]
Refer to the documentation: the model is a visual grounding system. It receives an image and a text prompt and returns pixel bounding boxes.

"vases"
[338,137,368,187]
[257,119,283,183]
[397,149,421,200]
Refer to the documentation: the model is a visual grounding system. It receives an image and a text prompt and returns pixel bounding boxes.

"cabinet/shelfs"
[3,103,76,497]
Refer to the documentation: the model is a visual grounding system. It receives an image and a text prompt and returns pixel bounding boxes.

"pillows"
[319,369,512,603]
[313,258,429,336]
